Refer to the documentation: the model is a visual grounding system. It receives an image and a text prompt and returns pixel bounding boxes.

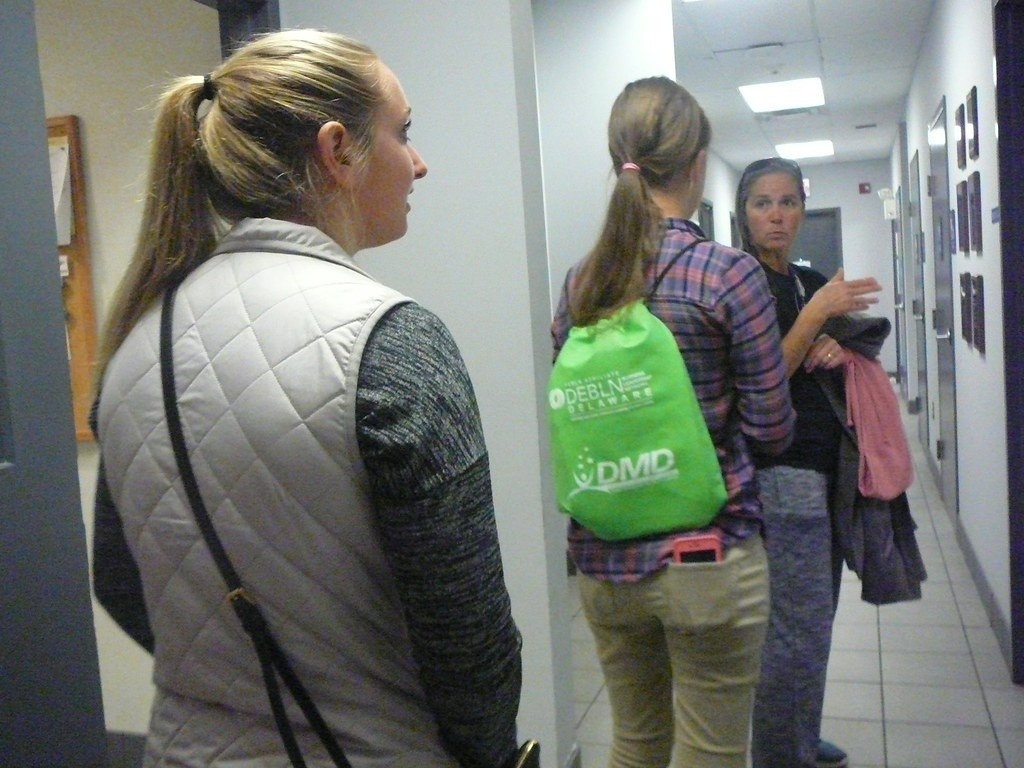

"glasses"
[740,158,803,194]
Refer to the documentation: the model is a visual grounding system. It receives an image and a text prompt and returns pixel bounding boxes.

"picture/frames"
[965,85,979,161]
[956,180,968,253]
[969,274,986,353]
[959,271,972,344]
[955,103,966,168]
[968,171,982,251]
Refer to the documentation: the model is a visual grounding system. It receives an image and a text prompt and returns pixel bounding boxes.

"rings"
[828,353,833,358]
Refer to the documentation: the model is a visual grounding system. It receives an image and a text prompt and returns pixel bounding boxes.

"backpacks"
[544,239,736,545]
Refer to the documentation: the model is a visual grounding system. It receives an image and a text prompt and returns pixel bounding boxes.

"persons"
[551,76,797,767]
[734,157,883,767]
[88,29,525,768]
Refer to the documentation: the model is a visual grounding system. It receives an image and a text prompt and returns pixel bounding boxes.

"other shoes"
[814,737,848,768]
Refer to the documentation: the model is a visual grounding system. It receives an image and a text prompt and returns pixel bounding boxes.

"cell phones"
[674,535,723,565]
